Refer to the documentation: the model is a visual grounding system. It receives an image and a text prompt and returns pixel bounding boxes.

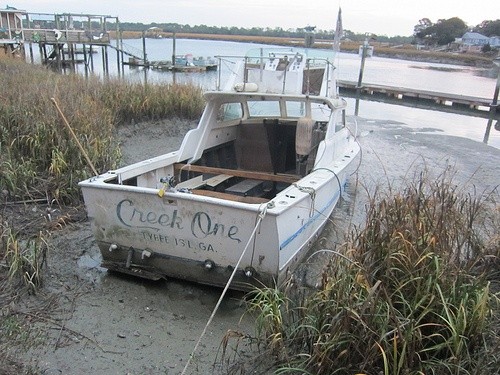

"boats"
[78,50,362,296]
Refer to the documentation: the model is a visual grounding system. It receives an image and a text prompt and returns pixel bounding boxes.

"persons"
[282,56,290,65]
